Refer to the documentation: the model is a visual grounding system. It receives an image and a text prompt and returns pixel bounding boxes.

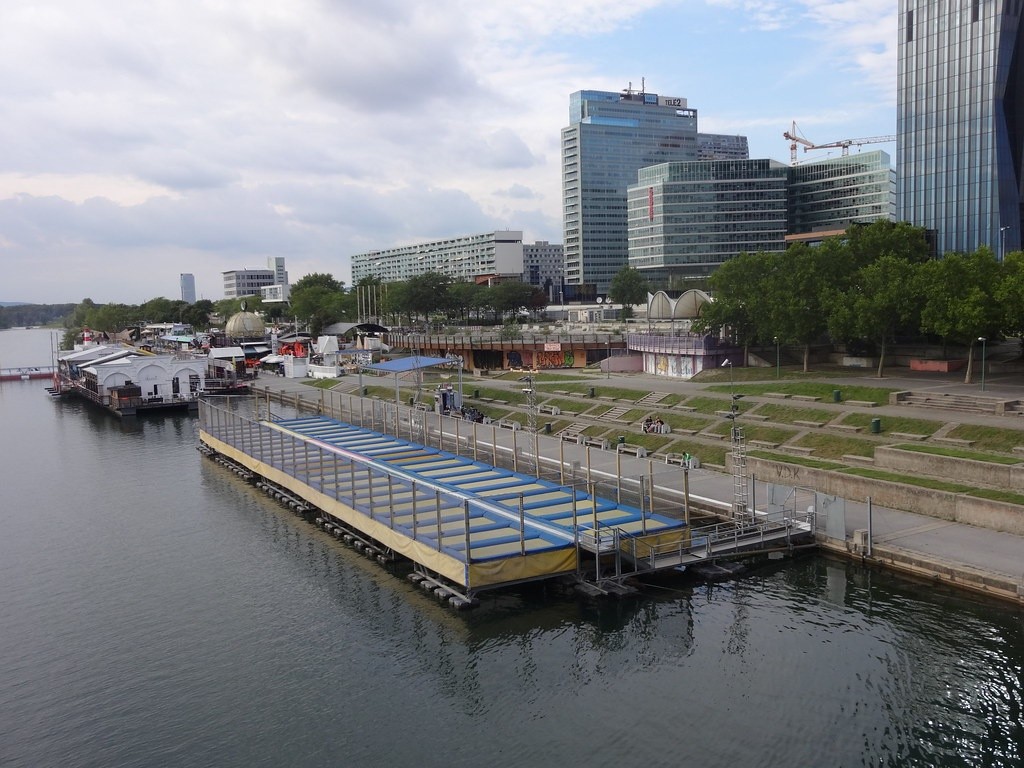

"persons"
[460,405,485,424]
[644,415,664,433]
[311,344,391,369]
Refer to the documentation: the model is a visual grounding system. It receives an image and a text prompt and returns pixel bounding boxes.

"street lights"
[977,337,989,394]
[773,336,779,379]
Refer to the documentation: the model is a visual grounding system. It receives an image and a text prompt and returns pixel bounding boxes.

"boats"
[0,365,60,381]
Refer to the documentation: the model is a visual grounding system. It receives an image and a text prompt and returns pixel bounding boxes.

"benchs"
[372,386,1023,473]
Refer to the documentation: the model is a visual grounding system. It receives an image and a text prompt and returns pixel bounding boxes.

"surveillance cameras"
[721,358,731,367]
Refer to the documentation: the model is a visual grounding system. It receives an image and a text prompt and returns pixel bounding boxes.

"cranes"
[783,119,815,164]
[802,133,895,158]
